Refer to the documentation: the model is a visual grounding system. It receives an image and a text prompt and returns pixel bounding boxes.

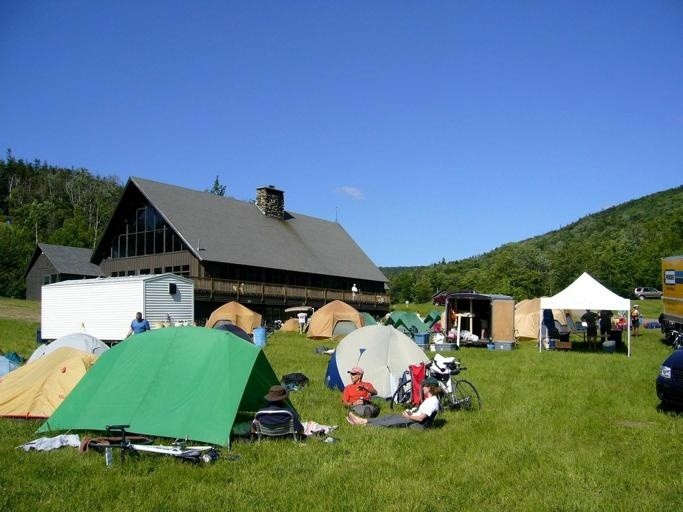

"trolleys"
[87,424,217,465]
[251,409,301,446]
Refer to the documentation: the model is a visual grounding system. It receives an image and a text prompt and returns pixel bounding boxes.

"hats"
[348,368,364,375]
[264,385,288,401]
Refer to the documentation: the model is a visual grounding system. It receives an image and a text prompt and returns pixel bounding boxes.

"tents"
[205,301,263,335]
[0,333,100,417]
[513,297,568,339]
[35,323,300,452]
[280,300,443,341]
[324,325,431,404]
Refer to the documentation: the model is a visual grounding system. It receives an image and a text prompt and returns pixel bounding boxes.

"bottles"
[104,447,113,466]
[360,396,365,404]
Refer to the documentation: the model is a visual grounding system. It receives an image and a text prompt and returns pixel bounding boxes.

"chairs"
[251,409,300,446]
[398,402,443,428]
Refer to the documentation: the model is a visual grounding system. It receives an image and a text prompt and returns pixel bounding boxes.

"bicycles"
[391,360,479,414]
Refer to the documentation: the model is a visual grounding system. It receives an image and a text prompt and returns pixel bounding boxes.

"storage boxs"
[412,332,430,344]
[433,343,457,351]
[492,341,516,352]
[416,343,430,350]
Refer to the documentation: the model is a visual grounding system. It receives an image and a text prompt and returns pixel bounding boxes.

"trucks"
[660,255,682,349]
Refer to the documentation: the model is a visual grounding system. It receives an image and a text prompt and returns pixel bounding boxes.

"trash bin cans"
[413,332,429,345]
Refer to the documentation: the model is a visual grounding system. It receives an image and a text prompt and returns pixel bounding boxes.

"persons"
[344,378,441,429]
[480,300,489,340]
[631,306,639,337]
[599,310,613,343]
[581,310,599,352]
[618,310,624,323]
[125,312,150,338]
[251,385,301,437]
[341,368,380,418]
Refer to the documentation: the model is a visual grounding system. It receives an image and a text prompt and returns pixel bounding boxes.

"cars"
[656,349,682,409]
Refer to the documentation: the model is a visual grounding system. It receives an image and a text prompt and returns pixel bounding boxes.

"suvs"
[632,286,664,300]
